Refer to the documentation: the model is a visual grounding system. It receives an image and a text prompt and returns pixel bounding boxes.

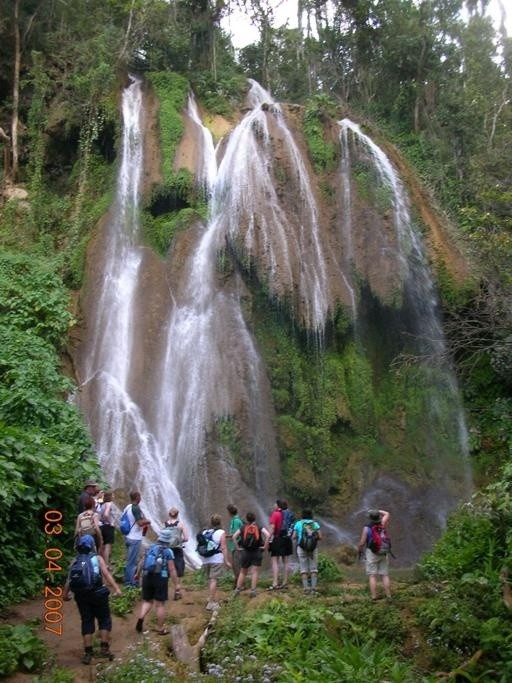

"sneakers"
[173,592,183,601]
[249,589,257,598]
[136,618,144,632]
[265,584,280,592]
[158,627,171,635]
[279,584,288,590]
[310,589,319,596]
[206,602,219,610]
[303,588,311,595]
[230,590,240,601]
[207,596,217,601]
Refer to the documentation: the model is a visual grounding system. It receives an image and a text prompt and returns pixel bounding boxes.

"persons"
[359,510,392,598]
[62,479,123,665]
[225,504,244,589]
[232,512,271,597]
[291,508,322,594]
[121,491,150,590]
[264,499,298,590]
[134,530,181,635]
[162,507,189,577]
[200,514,232,611]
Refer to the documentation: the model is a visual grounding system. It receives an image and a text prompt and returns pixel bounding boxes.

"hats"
[365,509,382,522]
[75,534,96,550]
[83,479,98,489]
[156,530,177,542]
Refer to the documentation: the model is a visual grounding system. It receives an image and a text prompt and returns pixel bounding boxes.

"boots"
[82,646,94,663]
[100,642,115,657]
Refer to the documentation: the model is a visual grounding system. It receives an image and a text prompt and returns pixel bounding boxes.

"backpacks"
[300,522,318,553]
[370,524,391,555]
[79,515,96,537]
[143,545,164,576]
[196,532,216,556]
[119,512,131,535]
[242,524,260,551]
[70,559,95,594]
[165,526,182,547]
[280,510,295,538]
